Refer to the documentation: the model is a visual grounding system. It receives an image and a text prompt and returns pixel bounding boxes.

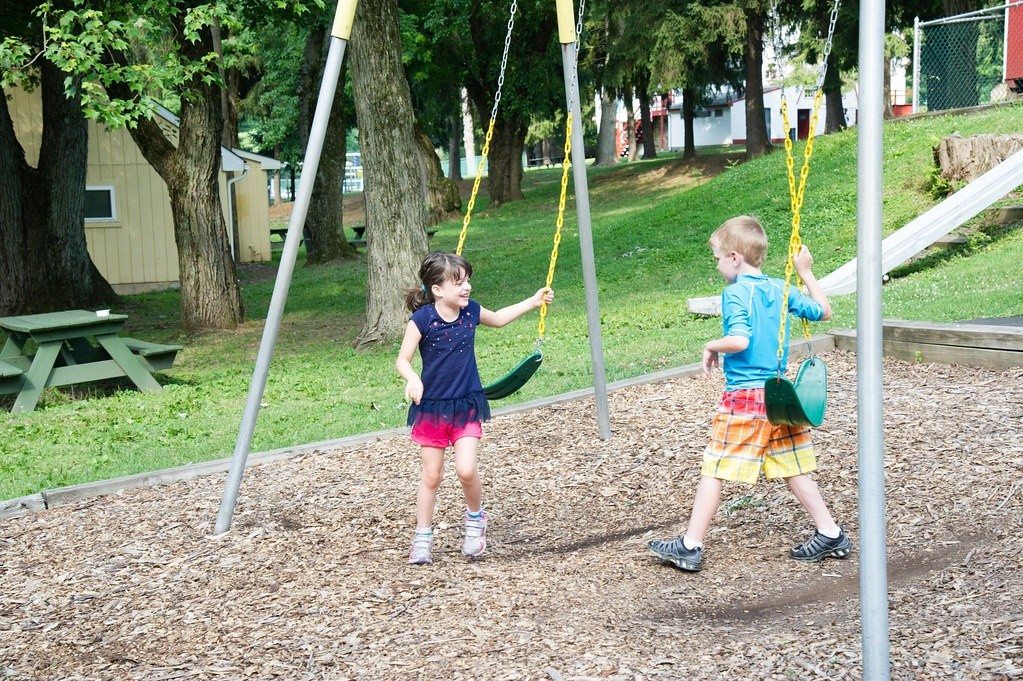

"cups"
[96,310,109,317]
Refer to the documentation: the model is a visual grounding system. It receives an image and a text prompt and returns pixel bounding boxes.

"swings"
[455,1,587,401]
[763,0,842,428]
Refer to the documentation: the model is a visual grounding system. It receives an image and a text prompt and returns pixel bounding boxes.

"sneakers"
[462,510,486,557]
[646,536,702,571]
[408,530,433,564]
[789,525,852,562]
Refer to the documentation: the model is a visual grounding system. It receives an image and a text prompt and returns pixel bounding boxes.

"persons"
[395,252,555,566]
[648,214,854,572]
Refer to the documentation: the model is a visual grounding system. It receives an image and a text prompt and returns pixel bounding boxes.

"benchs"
[348,230,439,248]
[0,337,185,396]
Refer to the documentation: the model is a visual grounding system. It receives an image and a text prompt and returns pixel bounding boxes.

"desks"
[347,224,365,239]
[0,308,162,413]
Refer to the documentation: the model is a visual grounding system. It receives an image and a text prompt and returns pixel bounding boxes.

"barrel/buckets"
[789,128,795,140]
